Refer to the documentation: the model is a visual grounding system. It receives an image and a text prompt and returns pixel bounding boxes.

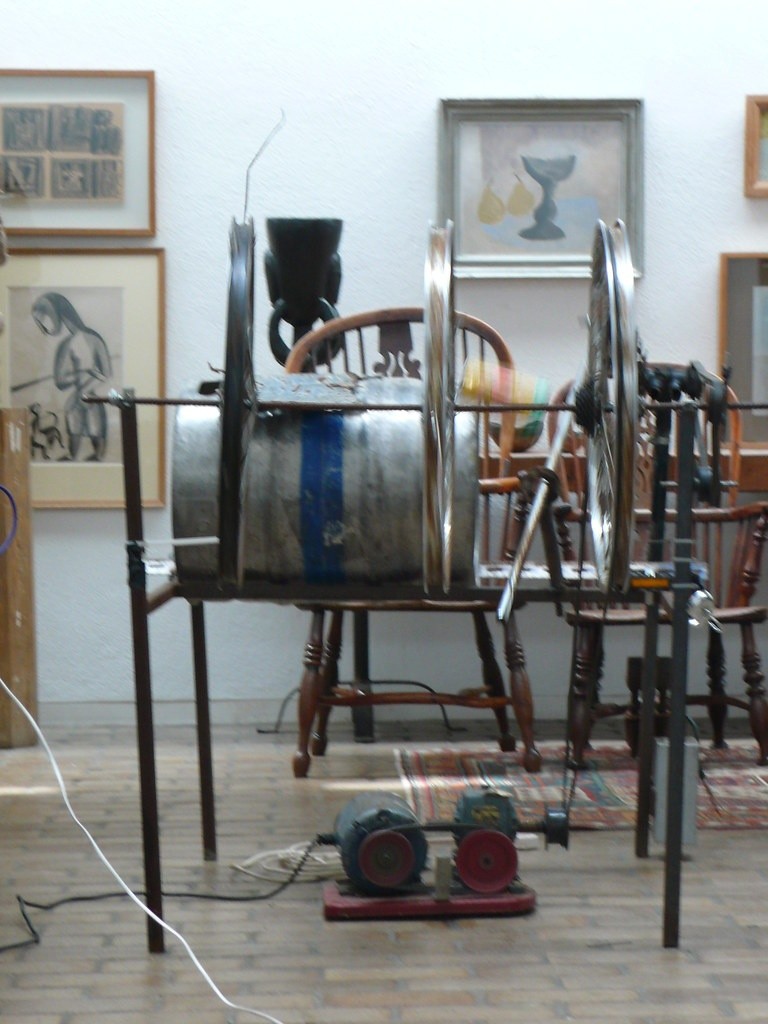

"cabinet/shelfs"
[0,409,34,746]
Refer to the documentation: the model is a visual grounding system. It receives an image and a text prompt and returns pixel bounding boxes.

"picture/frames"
[718,251,768,448]
[438,99,643,280]
[0,69,155,237]
[0,247,166,509]
[746,94,768,199]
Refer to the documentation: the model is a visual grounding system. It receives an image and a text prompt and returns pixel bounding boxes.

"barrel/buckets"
[169,374,478,597]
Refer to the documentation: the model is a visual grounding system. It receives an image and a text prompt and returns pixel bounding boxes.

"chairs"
[284,307,544,776]
[547,361,767,770]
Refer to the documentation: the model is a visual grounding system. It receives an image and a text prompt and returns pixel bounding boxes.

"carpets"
[395,741,768,830]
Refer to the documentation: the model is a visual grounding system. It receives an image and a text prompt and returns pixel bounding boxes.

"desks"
[351,448,767,744]
